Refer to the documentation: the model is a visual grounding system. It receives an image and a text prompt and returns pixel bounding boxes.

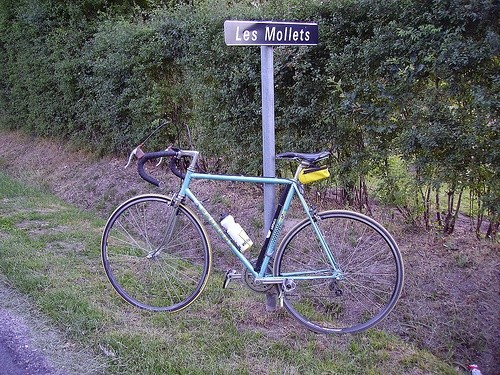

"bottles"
[218,213,254,251]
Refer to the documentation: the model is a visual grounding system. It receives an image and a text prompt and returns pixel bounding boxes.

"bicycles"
[100,119,403,336]
